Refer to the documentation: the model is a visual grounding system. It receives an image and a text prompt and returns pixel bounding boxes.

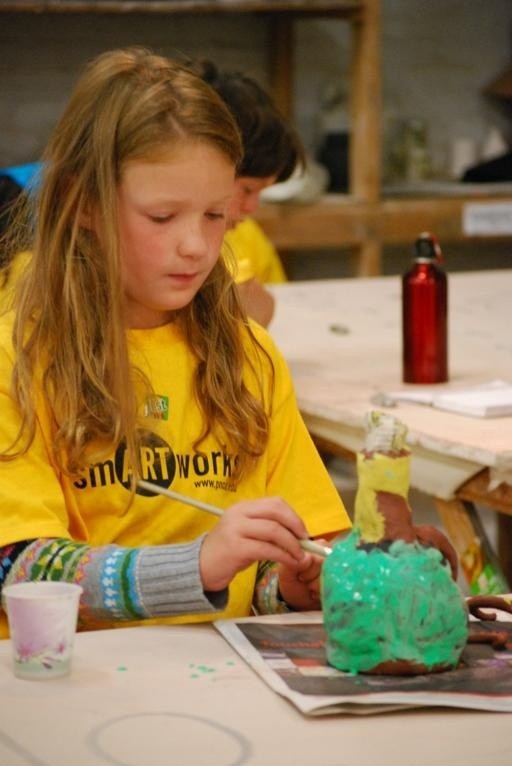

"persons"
[184,53,307,328]
[1,44,364,641]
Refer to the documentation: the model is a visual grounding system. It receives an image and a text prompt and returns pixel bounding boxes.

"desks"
[0,594,511,765]
[261,267,511,598]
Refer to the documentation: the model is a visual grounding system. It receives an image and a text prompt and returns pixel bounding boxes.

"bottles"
[402,231,449,383]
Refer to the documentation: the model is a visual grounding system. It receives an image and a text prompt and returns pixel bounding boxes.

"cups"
[2,582,84,679]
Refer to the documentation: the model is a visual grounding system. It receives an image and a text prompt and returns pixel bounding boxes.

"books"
[387,379,512,420]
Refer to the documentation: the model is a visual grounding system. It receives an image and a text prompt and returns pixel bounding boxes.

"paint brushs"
[136,479,332,557]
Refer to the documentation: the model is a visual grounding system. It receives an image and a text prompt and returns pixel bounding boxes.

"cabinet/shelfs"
[0,0,512,276]
